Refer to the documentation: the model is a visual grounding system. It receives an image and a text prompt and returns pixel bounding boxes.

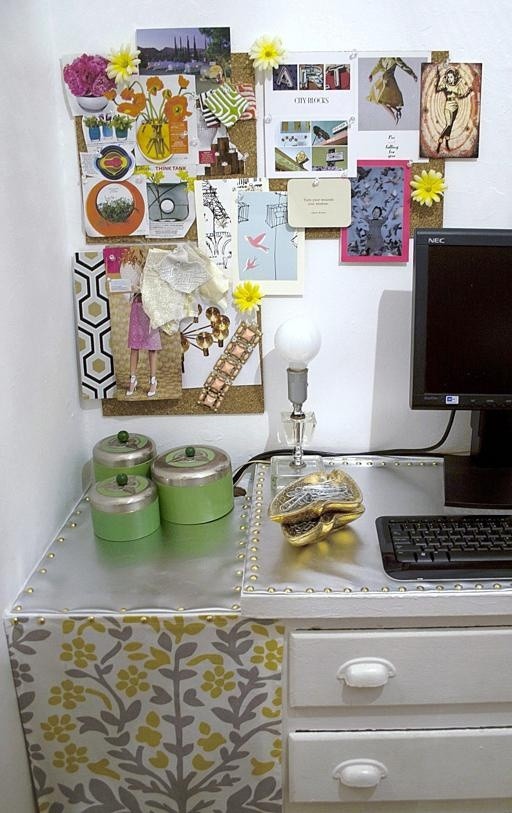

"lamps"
[271,317,324,491]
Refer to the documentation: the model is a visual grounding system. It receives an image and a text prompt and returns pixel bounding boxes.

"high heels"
[147,376,158,397]
[125,376,138,396]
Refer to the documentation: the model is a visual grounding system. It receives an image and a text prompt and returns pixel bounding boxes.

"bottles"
[90,432,234,540]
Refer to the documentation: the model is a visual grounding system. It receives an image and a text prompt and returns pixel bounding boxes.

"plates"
[269,468,367,547]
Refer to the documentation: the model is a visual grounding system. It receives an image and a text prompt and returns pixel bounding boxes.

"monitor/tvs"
[408,229,512,509]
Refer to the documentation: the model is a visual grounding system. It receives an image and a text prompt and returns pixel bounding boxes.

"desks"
[1,458,512,811]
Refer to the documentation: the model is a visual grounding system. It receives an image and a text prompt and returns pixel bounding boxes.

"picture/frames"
[340,161,410,263]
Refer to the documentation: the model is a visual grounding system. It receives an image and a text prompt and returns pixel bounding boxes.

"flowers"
[411,167,450,208]
[63,53,117,97]
[103,75,196,123]
[231,281,264,313]
[104,42,141,85]
[247,39,286,76]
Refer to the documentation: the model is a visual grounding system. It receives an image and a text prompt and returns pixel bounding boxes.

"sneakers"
[394,108,403,124]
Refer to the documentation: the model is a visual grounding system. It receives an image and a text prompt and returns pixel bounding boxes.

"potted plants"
[83,111,135,142]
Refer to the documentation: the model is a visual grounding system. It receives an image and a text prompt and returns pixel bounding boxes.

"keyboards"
[374,514,512,583]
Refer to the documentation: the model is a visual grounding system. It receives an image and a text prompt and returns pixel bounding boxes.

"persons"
[117,248,163,397]
[364,56,419,125]
[352,195,401,256]
[433,64,475,155]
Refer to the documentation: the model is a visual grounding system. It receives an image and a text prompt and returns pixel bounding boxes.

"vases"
[77,96,110,112]
[136,119,175,164]
